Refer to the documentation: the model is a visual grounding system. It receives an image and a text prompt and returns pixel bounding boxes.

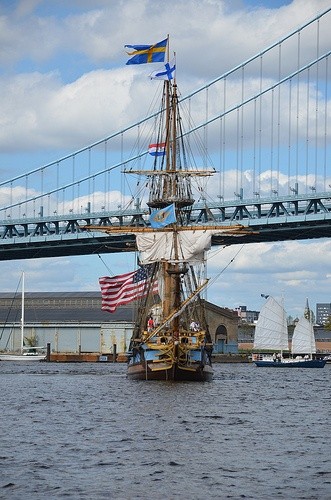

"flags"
[149,203,176,228]
[149,143,168,156]
[99,264,157,313]
[148,57,176,80]
[124,39,167,65]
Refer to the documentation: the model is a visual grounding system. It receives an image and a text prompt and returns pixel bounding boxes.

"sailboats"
[0,271,47,360]
[251,287,327,368]
[77,79,261,384]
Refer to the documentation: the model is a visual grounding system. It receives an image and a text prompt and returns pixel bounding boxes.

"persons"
[190,319,195,330]
[148,317,153,332]
